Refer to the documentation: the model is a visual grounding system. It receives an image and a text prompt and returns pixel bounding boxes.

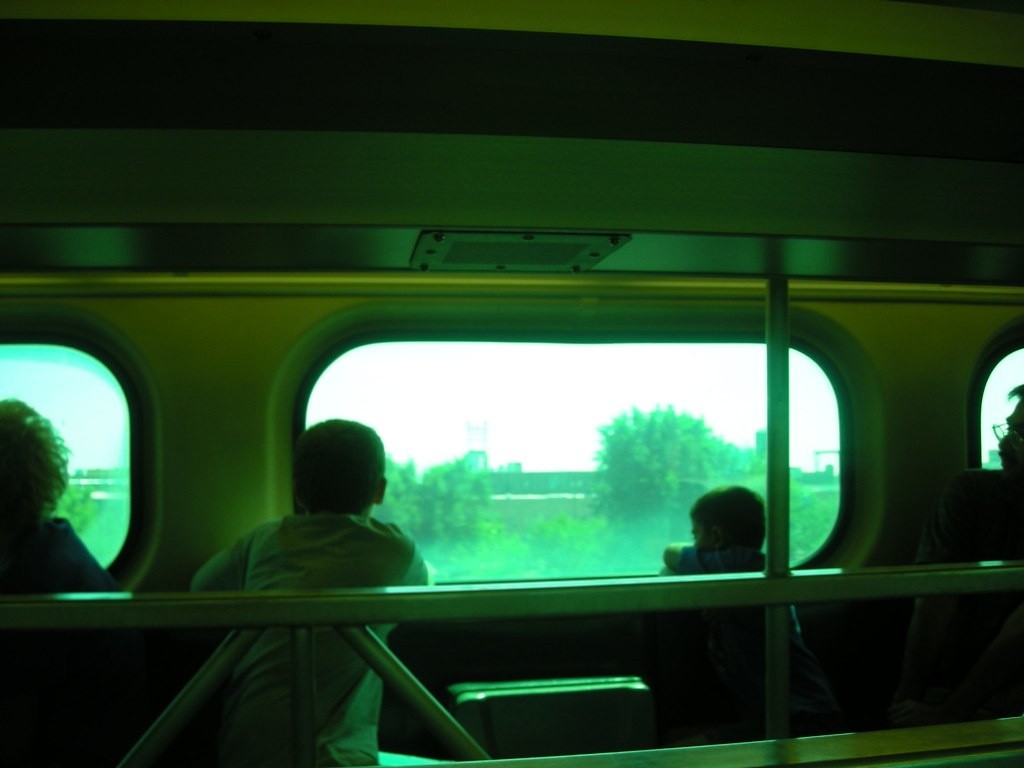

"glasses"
[993,423,1024,451]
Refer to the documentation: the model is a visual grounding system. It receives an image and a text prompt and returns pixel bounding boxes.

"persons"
[791,384,1024,724]
[641,489,852,741]
[191,421,412,768]
[1,401,123,768]
